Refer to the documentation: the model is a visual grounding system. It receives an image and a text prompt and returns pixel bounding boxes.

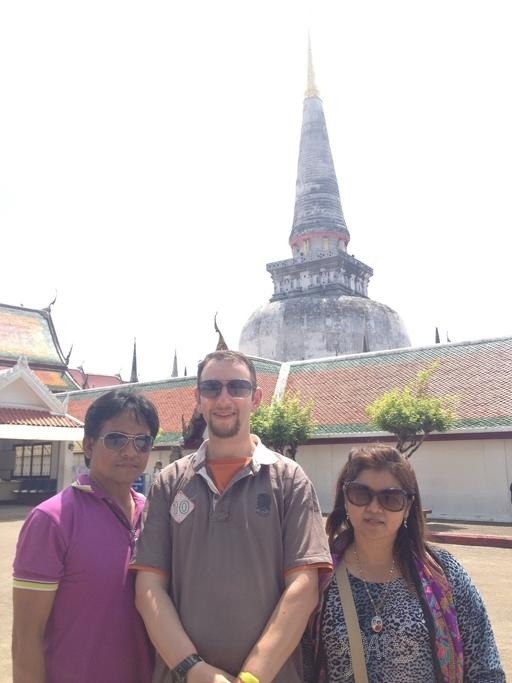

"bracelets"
[238,671,259,683]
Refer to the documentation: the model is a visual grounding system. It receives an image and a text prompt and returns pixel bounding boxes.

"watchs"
[170,653,202,683]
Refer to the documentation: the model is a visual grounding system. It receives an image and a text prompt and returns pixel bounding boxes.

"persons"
[12,389,159,683]
[128,350,334,683]
[302,446,507,682]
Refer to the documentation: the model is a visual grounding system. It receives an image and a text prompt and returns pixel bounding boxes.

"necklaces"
[352,542,395,633]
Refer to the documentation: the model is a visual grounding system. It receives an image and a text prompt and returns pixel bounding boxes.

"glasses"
[343,480,417,511]
[98,432,155,453]
[197,380,258,399]
